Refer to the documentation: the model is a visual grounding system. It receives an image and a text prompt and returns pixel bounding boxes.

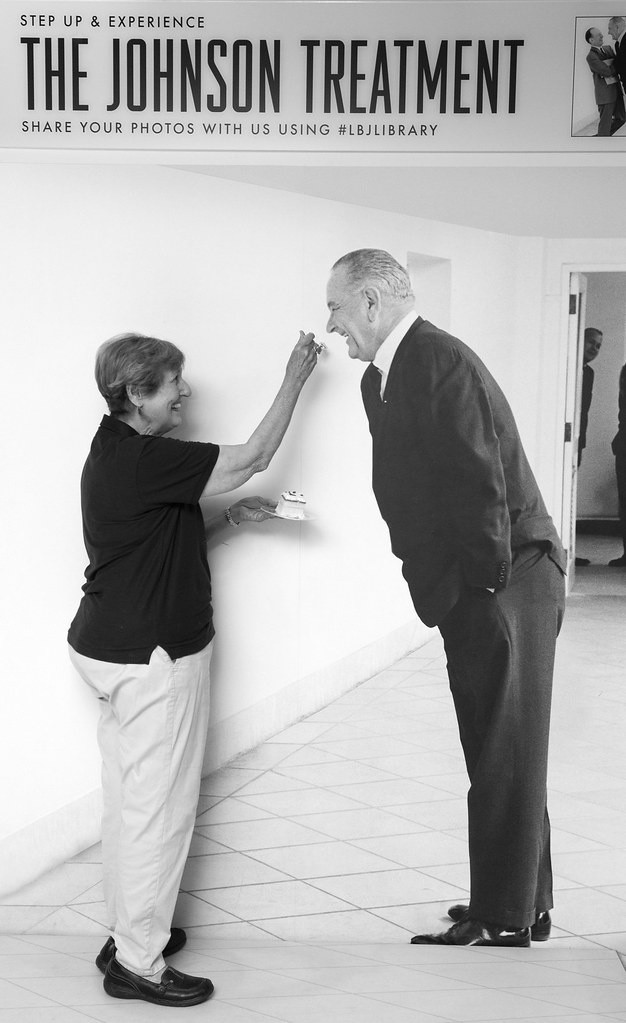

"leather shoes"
[411,912,532,947]
[447,904,551,941]
[103,953,214,1006]
[95,926,186,972]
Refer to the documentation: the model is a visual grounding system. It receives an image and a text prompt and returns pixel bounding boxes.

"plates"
[261,506,312,521]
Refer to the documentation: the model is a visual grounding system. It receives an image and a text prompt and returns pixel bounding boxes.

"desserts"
[275,490,306,519]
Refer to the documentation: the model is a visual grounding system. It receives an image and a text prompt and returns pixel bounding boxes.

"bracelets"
[224,505,241,529]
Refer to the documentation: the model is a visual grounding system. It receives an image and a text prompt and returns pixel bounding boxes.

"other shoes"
[573,557,591,566]
[609,552,626,567]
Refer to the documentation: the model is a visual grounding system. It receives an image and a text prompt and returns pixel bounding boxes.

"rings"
[312,347,317,353]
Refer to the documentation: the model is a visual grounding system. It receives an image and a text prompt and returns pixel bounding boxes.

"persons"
[576,17,626,138]
[574,328,626,567]
[326,248,567,948]
[66,329,326,1007]
